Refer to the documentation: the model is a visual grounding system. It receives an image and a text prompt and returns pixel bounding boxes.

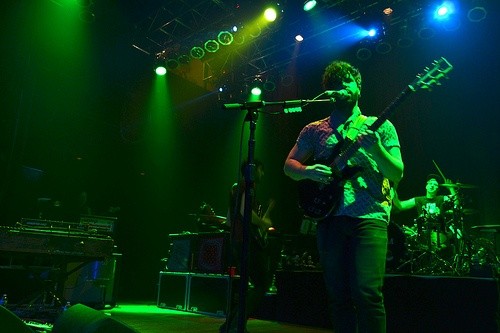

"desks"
[0,251,123,309]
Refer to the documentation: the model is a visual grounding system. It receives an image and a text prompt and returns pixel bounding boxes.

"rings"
[319,177,325,181]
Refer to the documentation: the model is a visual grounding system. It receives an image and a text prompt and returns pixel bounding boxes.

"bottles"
[64,301,71,312]
[0,294,8,307]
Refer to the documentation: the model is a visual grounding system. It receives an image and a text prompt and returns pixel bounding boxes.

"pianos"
[0,217,123,309]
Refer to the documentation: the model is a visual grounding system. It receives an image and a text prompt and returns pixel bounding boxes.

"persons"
[392,173,462,218]
[282,59,404,333]
[218,159,273,333]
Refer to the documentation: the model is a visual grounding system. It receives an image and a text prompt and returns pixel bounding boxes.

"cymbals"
[444,208,477,214]
[434,182,477,189]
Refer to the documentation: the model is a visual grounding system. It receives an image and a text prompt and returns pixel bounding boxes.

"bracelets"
[303,165,309,179]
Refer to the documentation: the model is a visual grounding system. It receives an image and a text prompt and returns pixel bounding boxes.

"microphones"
[325,89,348,101]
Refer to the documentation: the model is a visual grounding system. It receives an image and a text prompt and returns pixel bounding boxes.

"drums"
[385,221,406,272]
[416,215,448,244]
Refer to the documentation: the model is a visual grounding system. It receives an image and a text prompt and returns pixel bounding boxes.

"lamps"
[153,0,487,107]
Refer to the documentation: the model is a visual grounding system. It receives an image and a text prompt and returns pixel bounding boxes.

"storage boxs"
[157,271,241,318]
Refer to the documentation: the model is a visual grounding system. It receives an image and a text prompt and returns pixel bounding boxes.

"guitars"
[254,198,275,250]
[295,56,453,220]
